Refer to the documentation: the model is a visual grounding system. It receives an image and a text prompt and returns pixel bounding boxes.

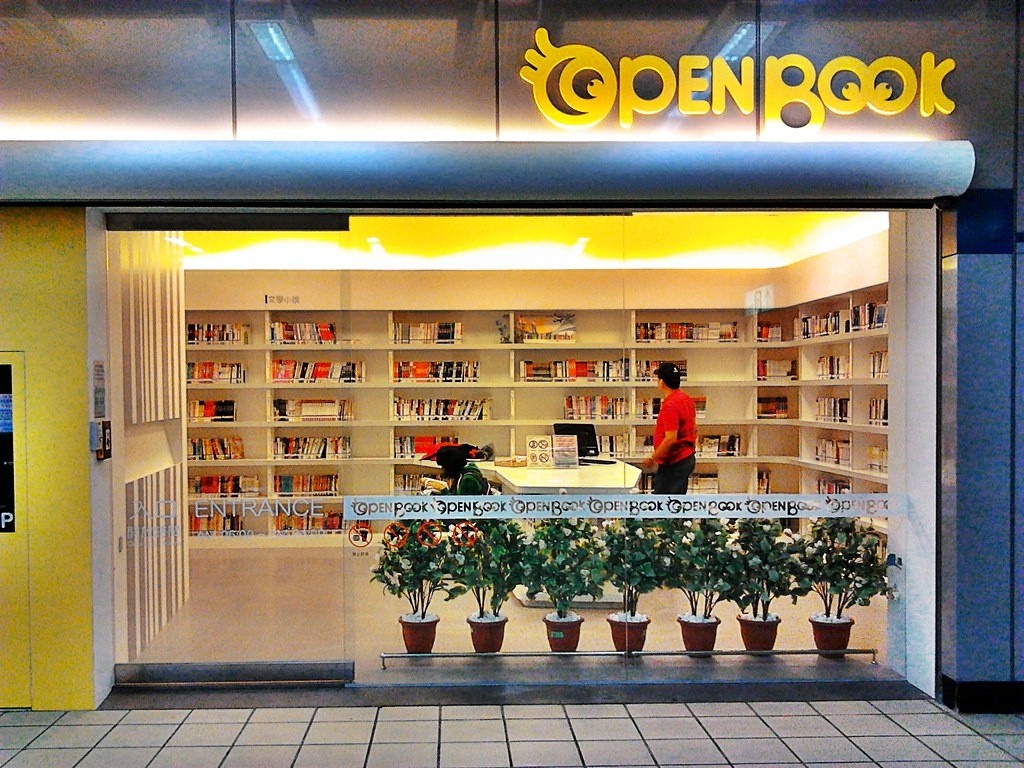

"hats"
[654,363,680,380]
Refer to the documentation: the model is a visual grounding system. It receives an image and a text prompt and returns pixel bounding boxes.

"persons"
[415,445,501,527]
[643,363,697,496]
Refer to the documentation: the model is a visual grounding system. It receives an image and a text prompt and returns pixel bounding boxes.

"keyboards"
[580,458,616,464]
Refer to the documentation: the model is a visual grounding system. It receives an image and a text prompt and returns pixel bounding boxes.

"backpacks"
[457,472,502,495]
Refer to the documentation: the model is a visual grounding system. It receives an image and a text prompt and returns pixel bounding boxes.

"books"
[853,300,890,521]
[271,322,371,536]
[390,320,496,497]
[756,319,799,494]
[518,359,630,460]
[186,321,259,536]
[803,307,852,496]
[634,321,741,495]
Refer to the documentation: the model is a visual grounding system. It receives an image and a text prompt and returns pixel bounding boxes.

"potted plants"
[519,518,603,653]
[727,517,792,653]
[442,512,527,653]
[678,519,732,657]
[783,519,889,657]
[597,520,681,653]
[370,492,456,653]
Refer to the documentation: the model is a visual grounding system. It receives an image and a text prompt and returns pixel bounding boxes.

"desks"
[415,456,644,603]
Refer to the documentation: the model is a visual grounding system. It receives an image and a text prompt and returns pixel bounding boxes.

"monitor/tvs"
[554,423,599,457]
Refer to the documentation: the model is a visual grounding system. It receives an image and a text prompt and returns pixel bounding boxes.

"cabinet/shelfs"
[186,282,891,572]
